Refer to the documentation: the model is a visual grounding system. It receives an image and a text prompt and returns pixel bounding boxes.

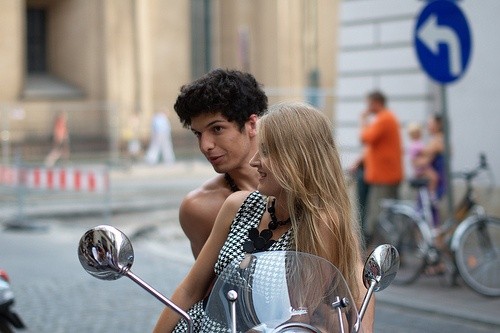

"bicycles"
[364,153,500,297]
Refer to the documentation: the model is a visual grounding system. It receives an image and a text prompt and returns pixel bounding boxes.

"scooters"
[78,224,401,333]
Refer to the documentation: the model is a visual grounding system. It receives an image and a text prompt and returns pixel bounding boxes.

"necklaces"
[224,172,241,193]
[244,197,302,252]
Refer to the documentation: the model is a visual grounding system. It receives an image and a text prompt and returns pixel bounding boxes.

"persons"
[153,102,360,333]
[412,112,452,277]
[42,110,70,167]
[173,69,375,333]
[145,107,176,167]
[348,91,404,268]
[405,122,445,206]
